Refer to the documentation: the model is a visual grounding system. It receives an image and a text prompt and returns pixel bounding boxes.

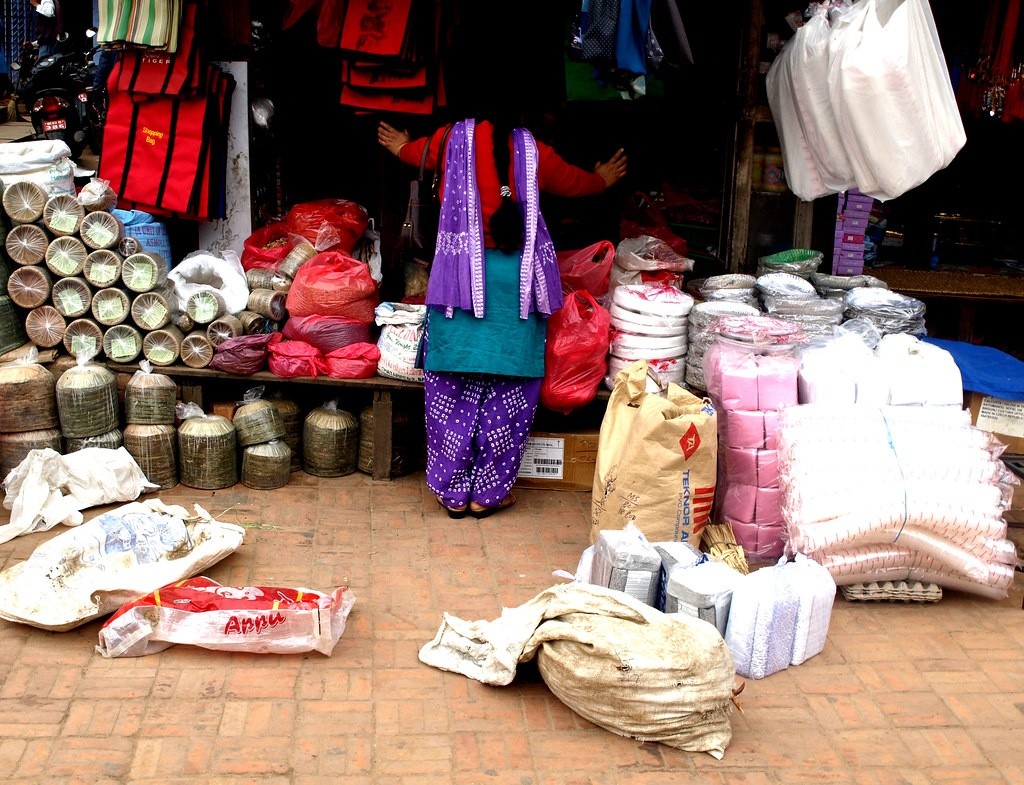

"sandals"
[470,491,516,519]
[436,492,468,519]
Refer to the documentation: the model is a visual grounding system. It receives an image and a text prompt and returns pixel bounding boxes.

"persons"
[378,60,628,517]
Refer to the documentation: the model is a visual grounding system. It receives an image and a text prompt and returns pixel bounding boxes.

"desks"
[47,350,426,483]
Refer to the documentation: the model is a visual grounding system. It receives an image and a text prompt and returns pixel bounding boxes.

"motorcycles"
[8,27,103,161]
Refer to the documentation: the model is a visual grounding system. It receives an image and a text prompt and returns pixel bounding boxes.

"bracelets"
[396,143,407,156]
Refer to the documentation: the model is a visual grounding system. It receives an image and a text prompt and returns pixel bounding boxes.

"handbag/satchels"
[561,0,701,111]
[400,122,456,251]
[209,198,381,375]
[620,191,687,257]
[555,240,614,300]
[538,290,610,415]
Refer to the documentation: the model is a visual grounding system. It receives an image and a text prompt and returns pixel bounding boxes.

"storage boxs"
[962,388,1024,527]
[510,432,598,492]
[591,531,836,679]
